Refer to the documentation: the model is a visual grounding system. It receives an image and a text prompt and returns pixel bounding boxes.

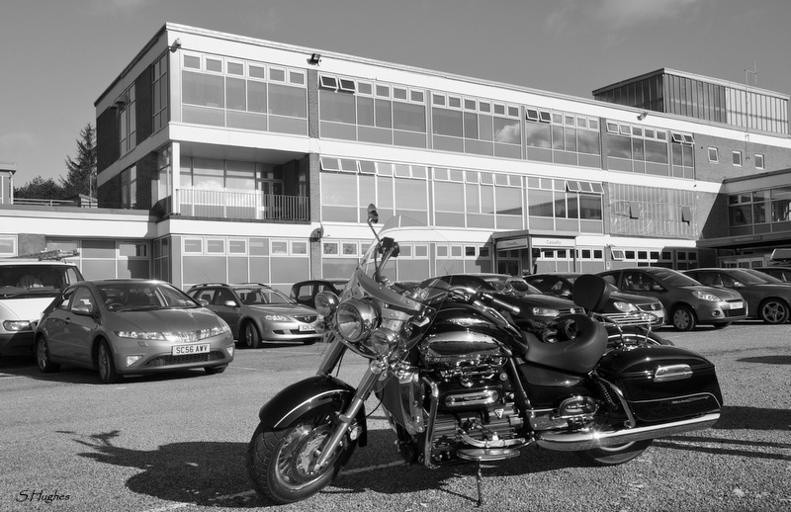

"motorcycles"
[247,203,724,505]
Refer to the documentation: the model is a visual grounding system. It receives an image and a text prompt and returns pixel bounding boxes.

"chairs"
[88,290,149,312]
[201,293,256,304]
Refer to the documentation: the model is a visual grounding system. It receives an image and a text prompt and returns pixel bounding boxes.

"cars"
[1,248,791,384]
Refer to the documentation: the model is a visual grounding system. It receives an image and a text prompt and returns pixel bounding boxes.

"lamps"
[311,54,321,63]
